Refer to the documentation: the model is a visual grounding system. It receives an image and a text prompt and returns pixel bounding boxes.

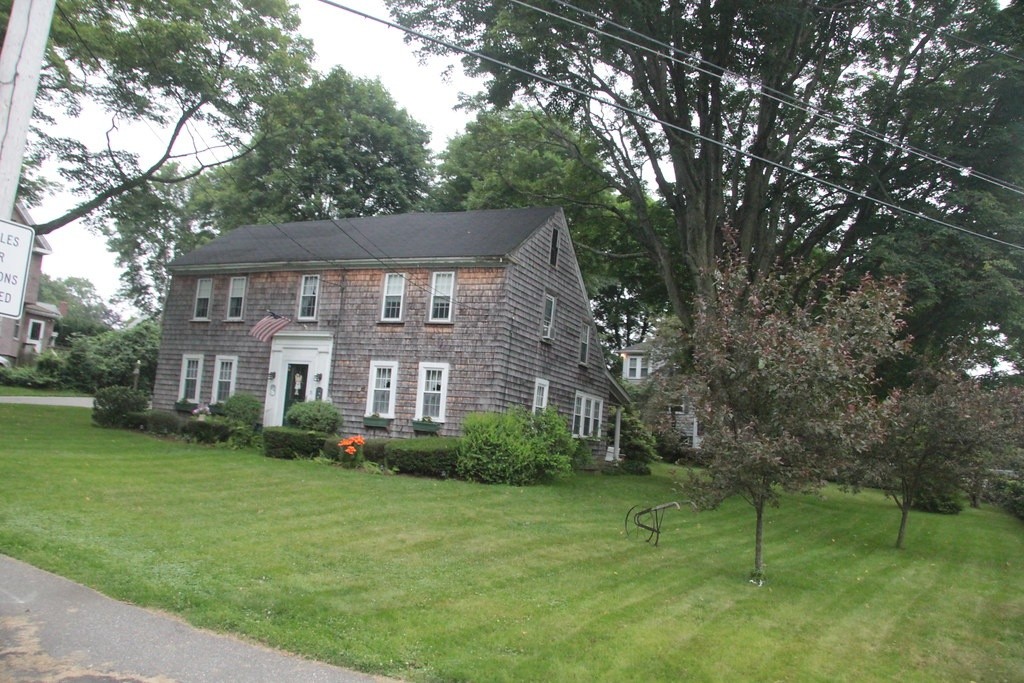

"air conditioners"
[543,325,556,341]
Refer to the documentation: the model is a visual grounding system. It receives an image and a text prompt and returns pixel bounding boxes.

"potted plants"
[174,397,199,411]
[363,413,394,428]
[412,416,441,432]
[208,401,225,414]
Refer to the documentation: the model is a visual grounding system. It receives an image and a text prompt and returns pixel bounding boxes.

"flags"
[247,310,291,342]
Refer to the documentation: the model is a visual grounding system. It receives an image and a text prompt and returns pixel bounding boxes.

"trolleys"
[624,499,698,546]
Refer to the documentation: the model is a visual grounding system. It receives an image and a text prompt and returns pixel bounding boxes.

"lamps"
[268,371,276,380]
[313,373,322,382]
